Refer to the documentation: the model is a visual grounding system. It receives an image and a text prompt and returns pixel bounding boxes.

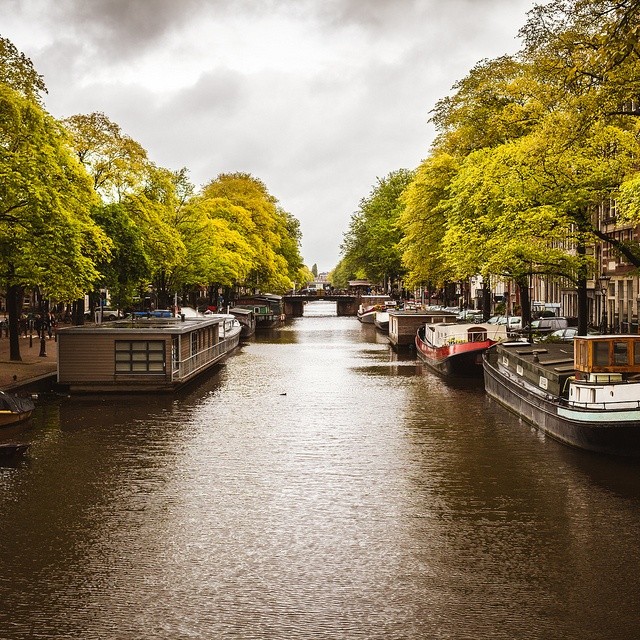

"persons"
[303,285,386,295]
[2,309,58,339]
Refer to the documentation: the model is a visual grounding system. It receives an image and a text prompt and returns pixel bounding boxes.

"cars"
[494,316,522,331]
[532,325,601,340]
[486,316,499,324]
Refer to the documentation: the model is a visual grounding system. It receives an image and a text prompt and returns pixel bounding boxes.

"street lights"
[39,285,47,357]
[597,275,611,335]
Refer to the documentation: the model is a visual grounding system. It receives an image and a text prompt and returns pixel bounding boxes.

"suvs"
[510,317,568,335]
[83,307,124,320]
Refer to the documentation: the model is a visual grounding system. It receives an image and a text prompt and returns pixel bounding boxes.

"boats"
[0,392,37,426]
[356,305,375,324]
[480,335,640,455]
[414,320,510,382]
